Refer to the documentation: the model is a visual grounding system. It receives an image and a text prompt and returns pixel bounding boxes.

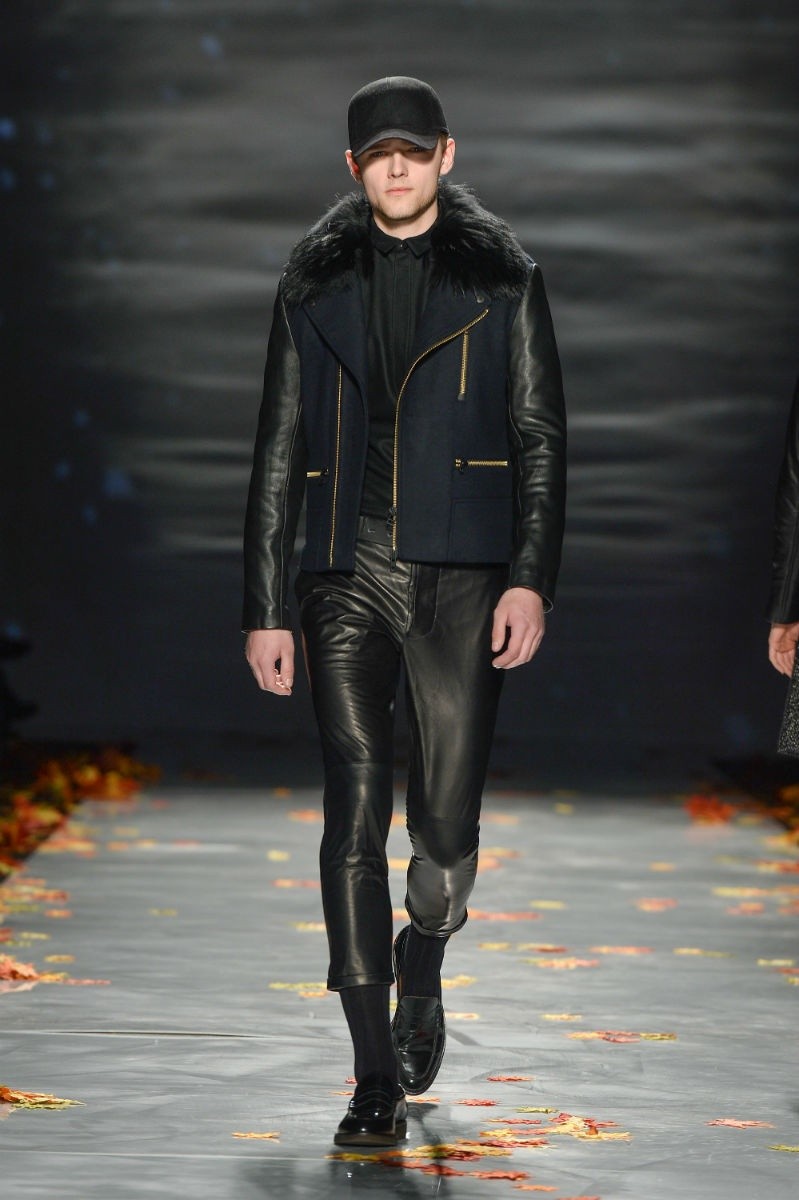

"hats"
[348,75,450,158]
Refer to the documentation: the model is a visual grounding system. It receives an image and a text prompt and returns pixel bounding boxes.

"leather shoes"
[390,924,447,1097]
[335,1079,410,1147]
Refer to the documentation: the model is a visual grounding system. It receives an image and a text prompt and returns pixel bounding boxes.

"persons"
[238,74,563,1145]
[768,372,798,761]
